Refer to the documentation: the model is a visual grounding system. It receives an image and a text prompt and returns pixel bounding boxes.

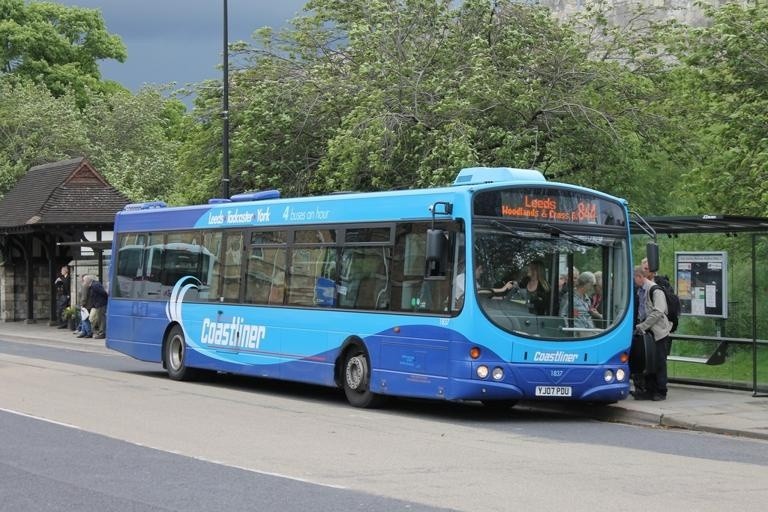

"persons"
[54,265,70,328]
[83,274,107,339]
[558,257,681,400]
[451,260,512,302]
[513,258,550,315]
[76,274,93,338]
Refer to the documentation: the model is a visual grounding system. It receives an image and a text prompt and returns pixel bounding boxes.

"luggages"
[629,326,659,377]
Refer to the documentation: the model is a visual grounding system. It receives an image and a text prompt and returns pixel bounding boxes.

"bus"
[106,167,660,415]
[117,242,215,303]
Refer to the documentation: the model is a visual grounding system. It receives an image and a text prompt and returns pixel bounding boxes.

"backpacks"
[651,275,681,334]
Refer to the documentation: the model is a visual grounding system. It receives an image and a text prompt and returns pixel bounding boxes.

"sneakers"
[629,389,668,402]
